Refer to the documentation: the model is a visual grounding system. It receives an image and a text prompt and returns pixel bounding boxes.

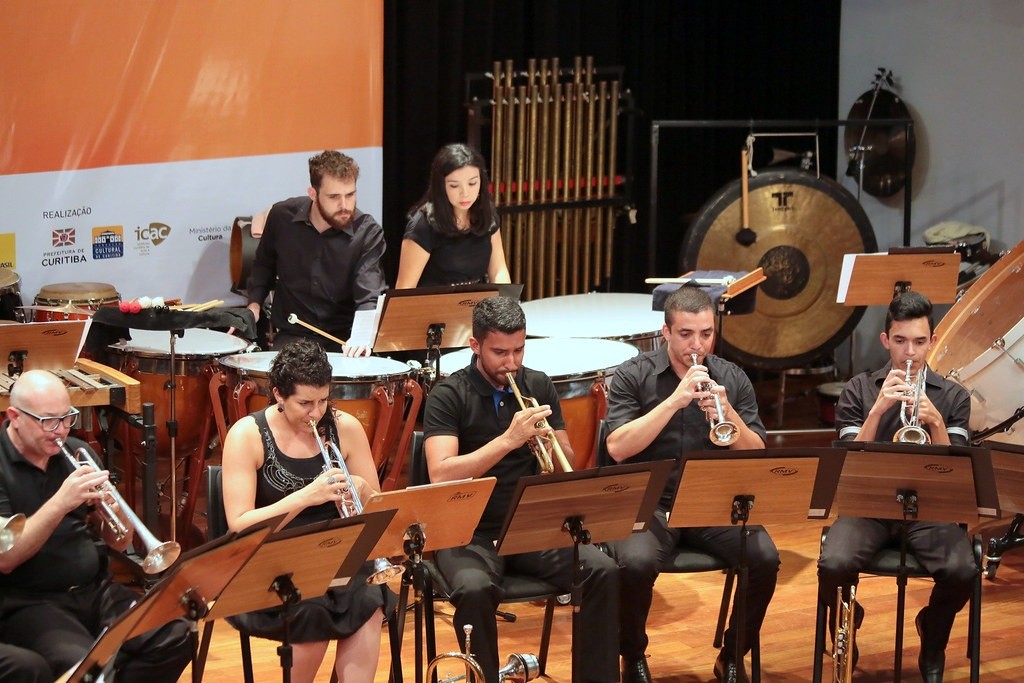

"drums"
[519,291,670,354]
[220,349,413,491]
[34,282,123,463]
[926,231,988,260]
[925,240,1023,445]
[107,327,255,450]
[0,267,26,319]
[427,338,642,472]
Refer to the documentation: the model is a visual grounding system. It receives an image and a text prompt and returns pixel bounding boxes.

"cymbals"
[844,90,916,198]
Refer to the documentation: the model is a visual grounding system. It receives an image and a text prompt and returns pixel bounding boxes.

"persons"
[818,291,980,683]
[223,340,384,683]
[603,287,781,683]
[396,143,511,289]
[227,150,386,358]
[423,297,621,683]
[0,369,194,683]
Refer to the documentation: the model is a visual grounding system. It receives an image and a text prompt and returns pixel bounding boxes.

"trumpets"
[832,584,857,683]
[892,357,931,446]
[56,435,182,575]
[505,369,577,473]
[425,623,486,682]
[690,352,739,447]
[309,418,406,584]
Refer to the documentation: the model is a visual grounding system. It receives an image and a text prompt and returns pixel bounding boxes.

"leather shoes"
[713,647,749,683]
[829,601,865,671]
[915,607,950,683]
[621,653,652,683]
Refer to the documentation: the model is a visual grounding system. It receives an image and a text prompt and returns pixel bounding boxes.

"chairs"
[599,420,736,649]
[813,514,984,682]
[397,432,576,682]
[229,217,277,346]
[194,465,399,683]
[75,508,149,605]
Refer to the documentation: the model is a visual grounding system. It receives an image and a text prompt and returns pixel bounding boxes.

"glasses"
[16,404,80,433]
